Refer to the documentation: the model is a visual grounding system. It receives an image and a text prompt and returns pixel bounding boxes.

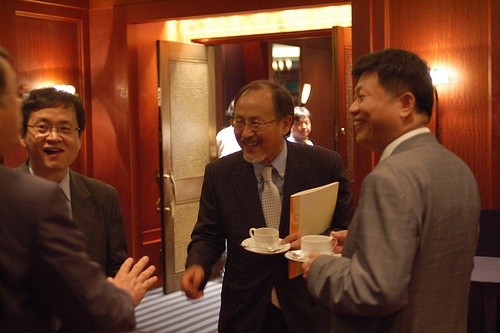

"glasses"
[27,121,80,136]
[228,118,278,131]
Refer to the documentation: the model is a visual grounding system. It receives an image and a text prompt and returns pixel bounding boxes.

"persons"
[15,87,128,279]
[287,106,313,145]
[208,99,242,161]
[181,80,355,333]
[0,50,158,332]
[299,48,480,333]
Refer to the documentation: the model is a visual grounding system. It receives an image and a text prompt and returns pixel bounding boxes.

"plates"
[241,238,291,254]
[284,250,341,262]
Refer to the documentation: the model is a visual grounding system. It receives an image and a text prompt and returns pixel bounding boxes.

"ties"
[261,166,281,230]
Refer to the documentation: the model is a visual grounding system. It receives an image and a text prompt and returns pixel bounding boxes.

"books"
[288,182,339,278]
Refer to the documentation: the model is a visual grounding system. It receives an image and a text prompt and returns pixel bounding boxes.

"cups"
[249,228,279,249]
[302,235,337,259]
